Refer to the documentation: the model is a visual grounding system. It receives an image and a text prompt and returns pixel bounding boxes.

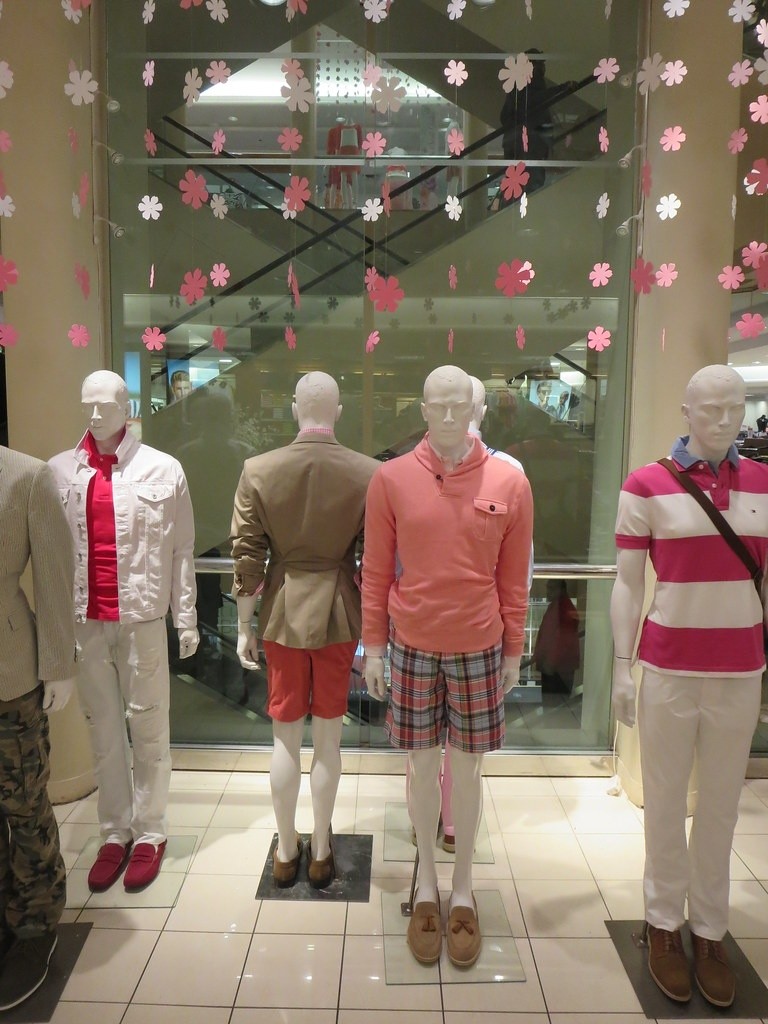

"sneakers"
[691,928,734,1007]
[646,923,692,1003]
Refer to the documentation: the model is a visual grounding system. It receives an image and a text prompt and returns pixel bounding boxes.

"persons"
[228,371,381,887]
[49,370,198,892]
[498,47,580,211]
[195,547,224,660]
[755,414,768,438]
[536,381,569,421]
[0,445,68,1008]
[611,363,768,1008]
[365,365,534,969]
[534,579,580,729]
[170,371,192,401]
[323,117,462,211]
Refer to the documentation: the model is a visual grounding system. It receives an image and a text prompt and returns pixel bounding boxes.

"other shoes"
[442,835,455,853]
[273,830,303,888]
[88,836,135,892]
[411,812,444,845]
[123,838,168,888]
[447,889,482,966]
[307,837,334,889]
[0,931,59,1010]
[408,885,442,963]
